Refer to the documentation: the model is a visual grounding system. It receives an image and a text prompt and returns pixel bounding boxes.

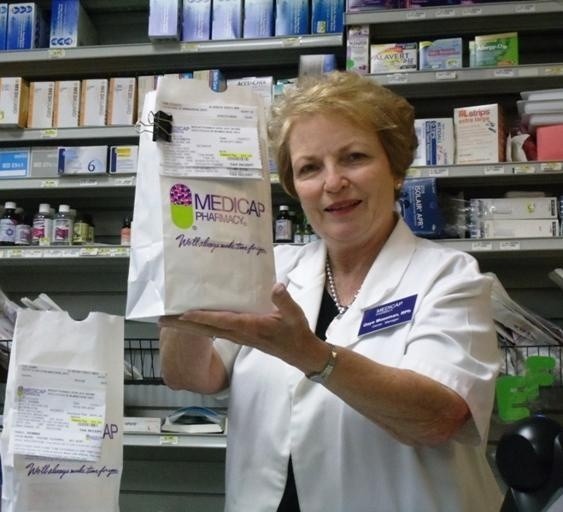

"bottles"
[119,217,130,246]
[0,200,95,246]
[274,204,318,245]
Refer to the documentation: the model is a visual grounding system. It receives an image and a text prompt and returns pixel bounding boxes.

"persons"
[158,69,511,512]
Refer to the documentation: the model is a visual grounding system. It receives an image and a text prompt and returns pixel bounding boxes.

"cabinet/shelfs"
[0,28,345,451]
[342,0,563,444]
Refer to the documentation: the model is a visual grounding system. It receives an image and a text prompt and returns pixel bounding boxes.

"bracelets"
[302,343,338,383]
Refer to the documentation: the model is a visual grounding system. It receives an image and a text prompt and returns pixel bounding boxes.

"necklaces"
[325,257,364,315]
